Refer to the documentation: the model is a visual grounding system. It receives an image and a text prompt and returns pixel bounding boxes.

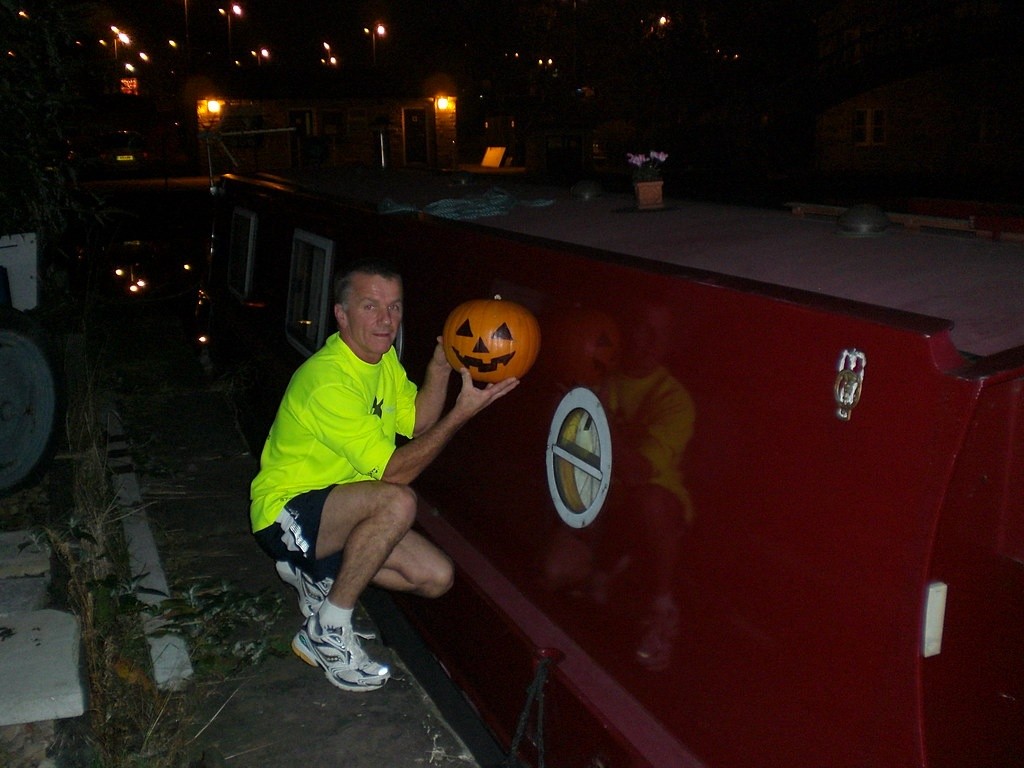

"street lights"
[227,2,243,61]
[372,22,386,68]
[110,25,120,60]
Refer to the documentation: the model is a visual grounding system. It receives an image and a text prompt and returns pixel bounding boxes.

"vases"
[634,181,666,211]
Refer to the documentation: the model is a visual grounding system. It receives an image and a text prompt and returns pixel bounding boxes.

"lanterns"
[444,294,541,384]
[541,302,620,382]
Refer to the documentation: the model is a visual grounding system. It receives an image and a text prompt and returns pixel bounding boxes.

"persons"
[250,260,521,692]
[537,306,696,666]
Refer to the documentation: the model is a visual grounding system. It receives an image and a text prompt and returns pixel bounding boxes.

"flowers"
[625,151,670,184]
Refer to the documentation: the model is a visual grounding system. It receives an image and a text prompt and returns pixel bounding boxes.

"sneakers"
[276,554,337,617]
[292,612,390,692]
